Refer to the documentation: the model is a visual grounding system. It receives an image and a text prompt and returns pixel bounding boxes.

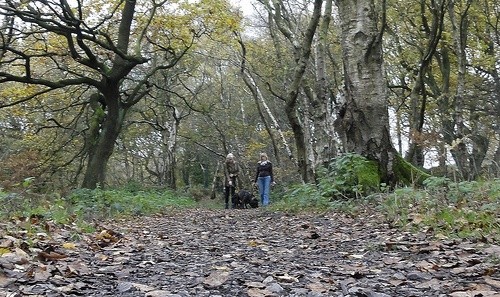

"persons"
[252,152,274,206]
[223,153,240,209]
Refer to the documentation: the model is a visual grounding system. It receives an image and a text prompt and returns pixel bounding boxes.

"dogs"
[234,190,258,209]
